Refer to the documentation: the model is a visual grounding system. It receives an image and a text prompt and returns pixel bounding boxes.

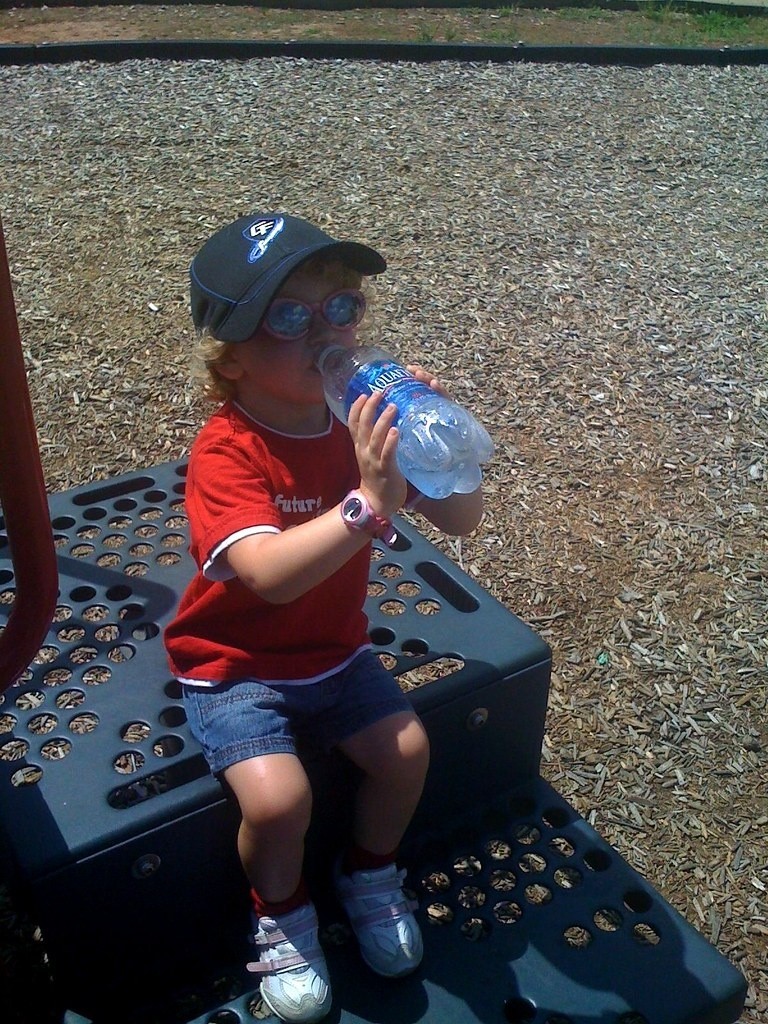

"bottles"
[310,339,496,499]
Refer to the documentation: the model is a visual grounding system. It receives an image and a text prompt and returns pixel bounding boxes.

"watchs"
[341,488,413,551]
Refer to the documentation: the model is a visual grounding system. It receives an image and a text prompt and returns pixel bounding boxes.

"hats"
[187,212,388,343]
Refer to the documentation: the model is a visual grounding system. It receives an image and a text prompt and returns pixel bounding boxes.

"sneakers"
[335,862,424,980]
[246,903,334,1024]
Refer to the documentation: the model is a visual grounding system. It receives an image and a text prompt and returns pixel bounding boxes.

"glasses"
[261,290,367,341]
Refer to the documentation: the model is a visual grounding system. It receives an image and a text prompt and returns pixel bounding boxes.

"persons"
[164,214,481,1024]
